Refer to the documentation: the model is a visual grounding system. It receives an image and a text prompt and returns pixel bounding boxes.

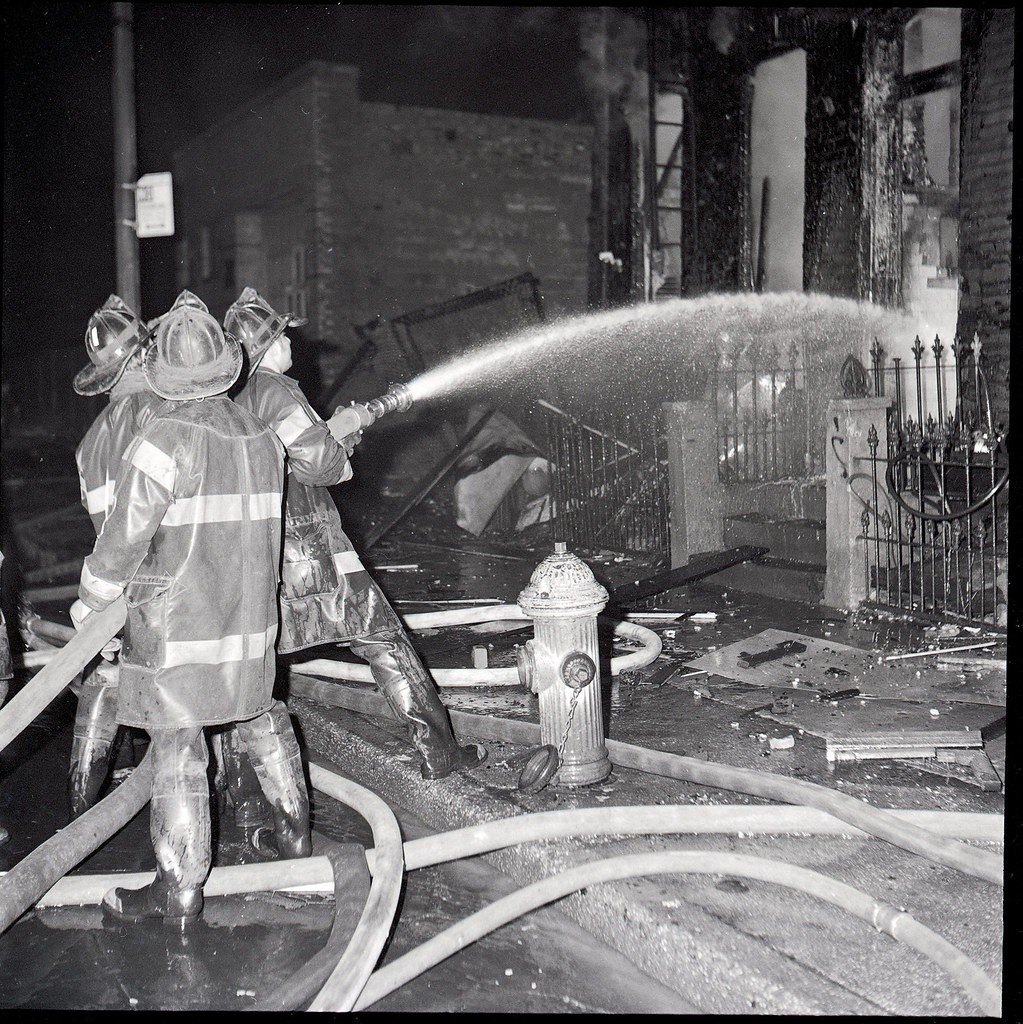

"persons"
[65,294,485,925]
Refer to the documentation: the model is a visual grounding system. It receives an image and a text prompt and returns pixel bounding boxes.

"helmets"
[72,294,160,396]
[224,287,310,380]
[142,289,243,402]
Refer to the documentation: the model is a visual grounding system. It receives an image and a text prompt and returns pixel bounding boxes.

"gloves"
[69,599,121,661]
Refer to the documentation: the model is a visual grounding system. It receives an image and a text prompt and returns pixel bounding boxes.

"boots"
[236,701,312,860]
[69,654,119,821]
[102,726,211,926]
[221,723,275,827]
[351,629,488,779]
